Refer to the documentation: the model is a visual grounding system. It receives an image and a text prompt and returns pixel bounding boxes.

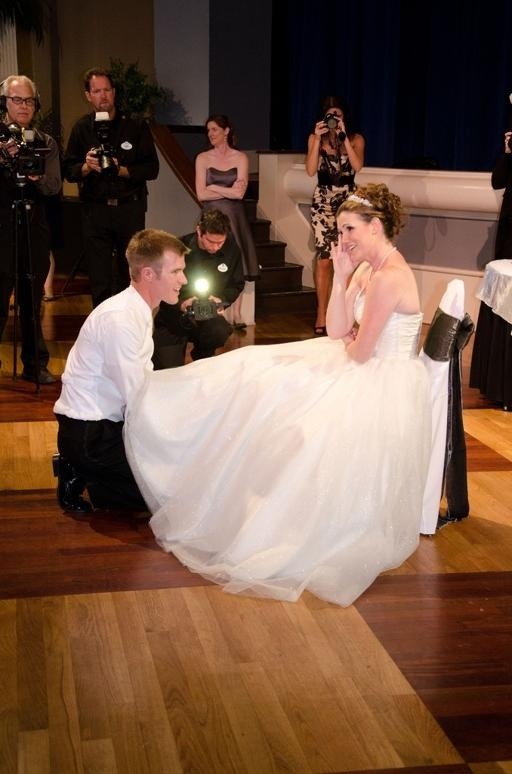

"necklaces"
[368,246,396,284]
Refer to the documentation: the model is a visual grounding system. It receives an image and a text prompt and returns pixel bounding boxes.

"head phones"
[0,80,41,114]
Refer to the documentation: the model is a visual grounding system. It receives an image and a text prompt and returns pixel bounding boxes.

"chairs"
[416,279,468,536]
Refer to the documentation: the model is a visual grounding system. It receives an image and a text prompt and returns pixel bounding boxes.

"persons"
[195,113,261,329]
[124,183,433,607]
[51,228,191,513]
[65,66,159,308]
[305,94,366,334]
[151,207,244,370]
[468,92,511,406]
[1,74,64,384]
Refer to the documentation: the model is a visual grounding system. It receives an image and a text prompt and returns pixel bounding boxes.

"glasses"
[8,96,36,106]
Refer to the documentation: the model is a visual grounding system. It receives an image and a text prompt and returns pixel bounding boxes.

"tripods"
[12,182,41,397]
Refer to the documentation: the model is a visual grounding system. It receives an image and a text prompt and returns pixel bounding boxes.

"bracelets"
[331,282,346,293]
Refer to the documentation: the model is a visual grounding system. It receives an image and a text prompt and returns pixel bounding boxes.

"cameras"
[324,113,339,129]
[95,112,118,182]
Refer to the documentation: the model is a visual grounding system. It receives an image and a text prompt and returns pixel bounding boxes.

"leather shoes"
[22,369,55,383]
[53,454,92,514]
